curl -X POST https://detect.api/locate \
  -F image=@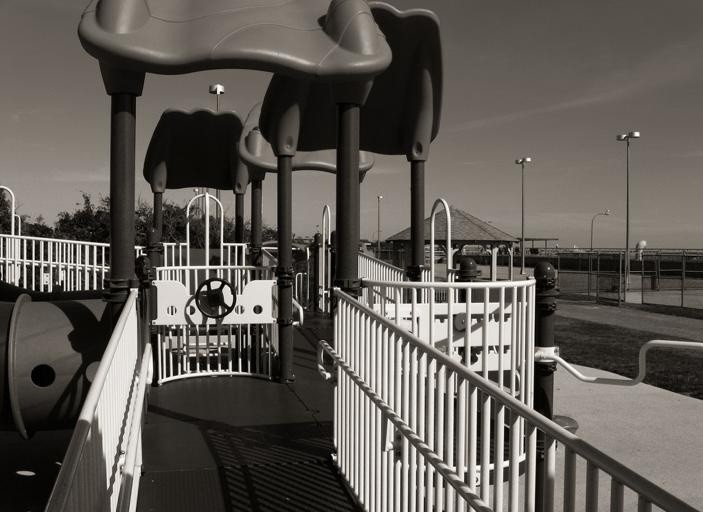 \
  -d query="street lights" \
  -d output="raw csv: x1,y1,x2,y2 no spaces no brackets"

615,131,640,251
589,208,612,251
514,158,533,273
376,195,383,257
192,189,198,216
208,83,224,113
